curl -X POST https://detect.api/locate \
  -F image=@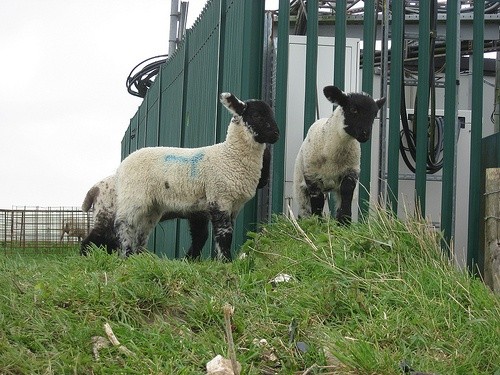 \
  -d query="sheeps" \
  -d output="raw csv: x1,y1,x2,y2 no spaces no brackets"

294,85,387,226
79,91,280,259
